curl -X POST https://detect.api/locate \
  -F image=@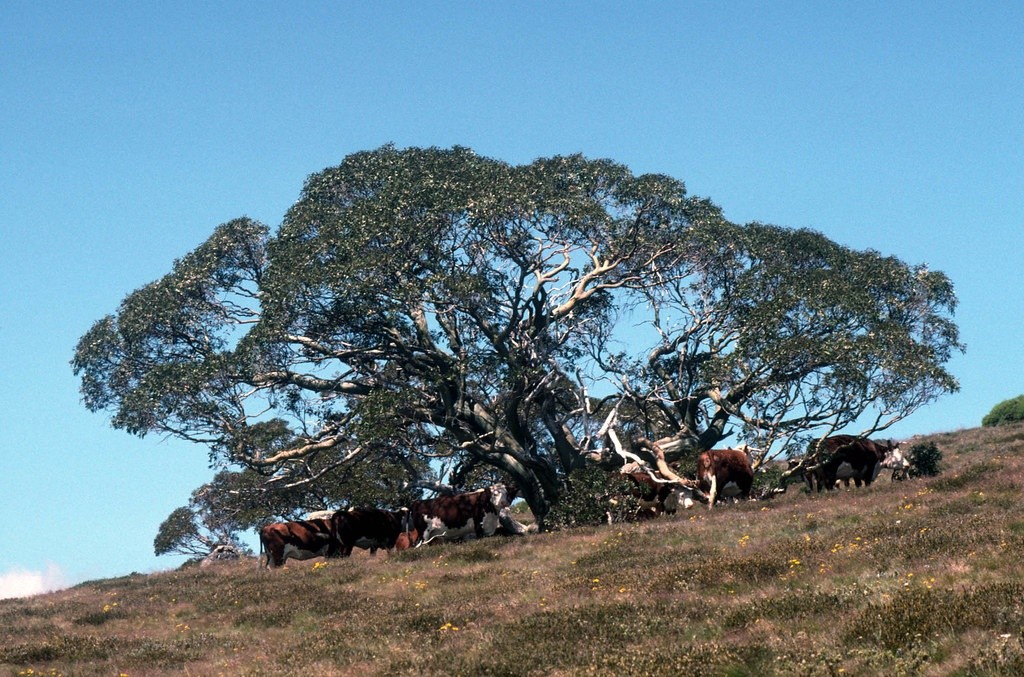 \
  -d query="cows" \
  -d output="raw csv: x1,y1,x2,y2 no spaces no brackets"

258,434,911,572
